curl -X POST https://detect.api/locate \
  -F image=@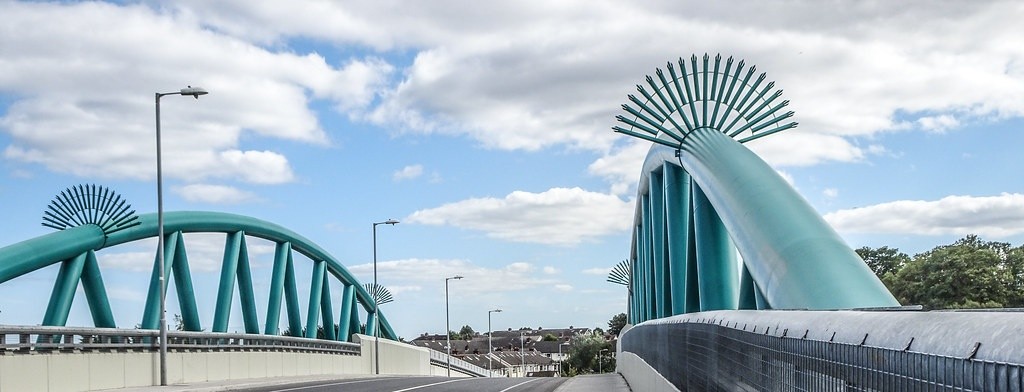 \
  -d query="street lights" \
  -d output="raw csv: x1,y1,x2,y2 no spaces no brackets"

489,309,502,379
559,343,566,376
154,85,209,386
446,276,464,377
520,330,530,377
600,348,609,374
372,219,400,375
